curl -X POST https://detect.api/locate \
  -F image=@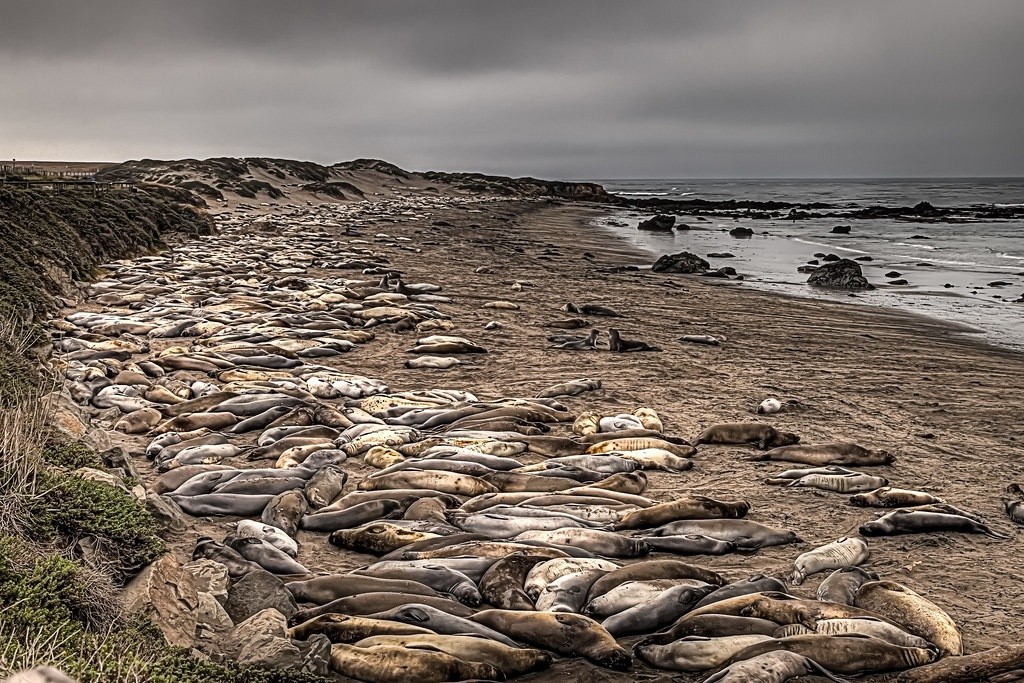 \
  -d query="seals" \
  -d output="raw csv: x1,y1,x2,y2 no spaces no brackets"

37,184,1024,683
